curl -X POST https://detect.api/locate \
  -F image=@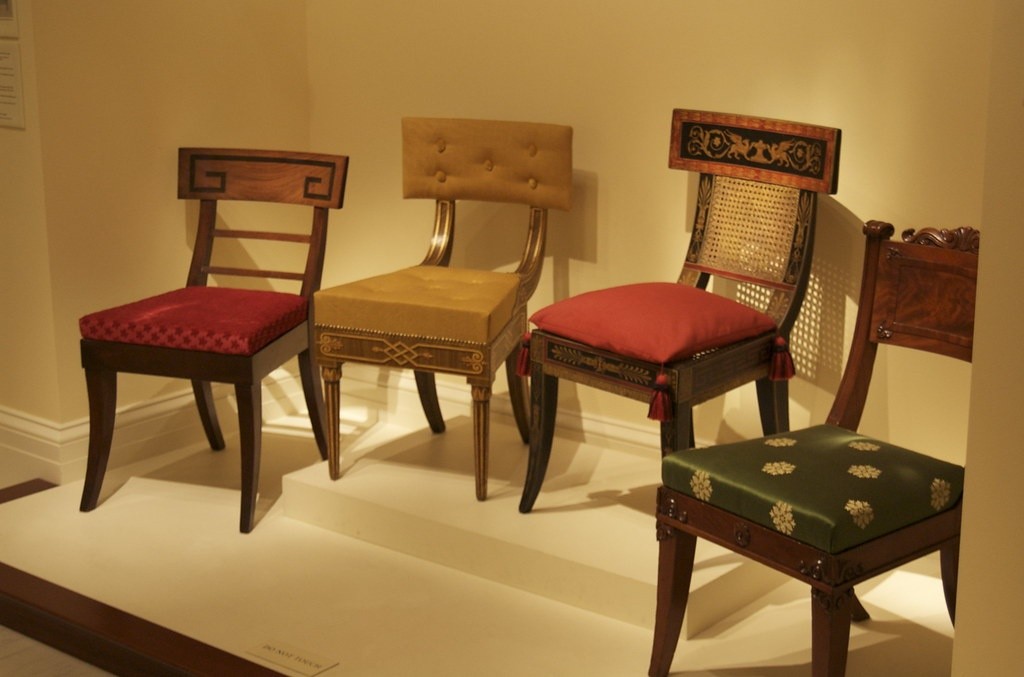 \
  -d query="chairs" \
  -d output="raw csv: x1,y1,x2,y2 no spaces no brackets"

517,108,843,513
312,119,572,501
645,219,981,677
77,149,350,534
